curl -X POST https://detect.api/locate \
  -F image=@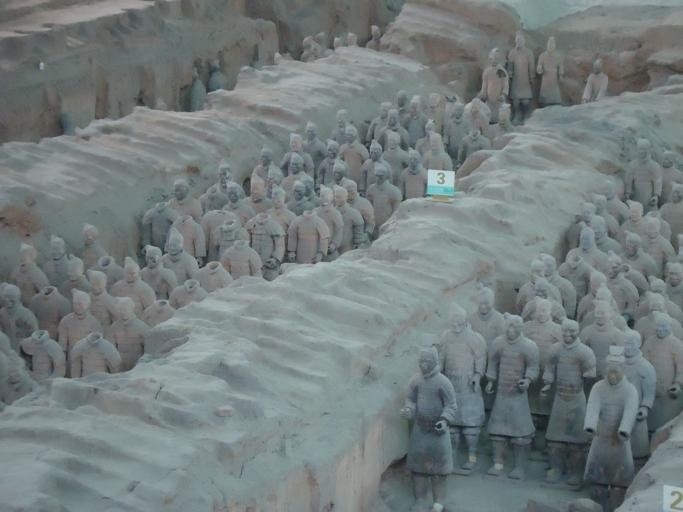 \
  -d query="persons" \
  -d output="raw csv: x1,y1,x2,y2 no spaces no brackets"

0,25,609,408
403,138,682,512
